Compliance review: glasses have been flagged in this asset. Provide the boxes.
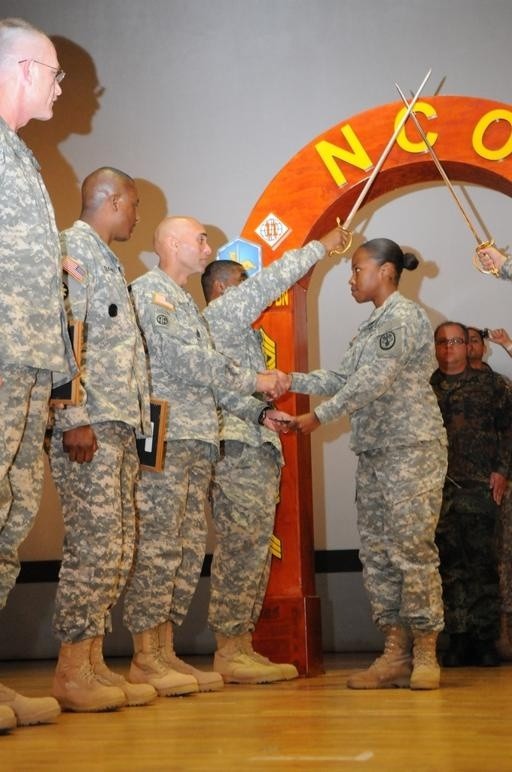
[436,337,466,346]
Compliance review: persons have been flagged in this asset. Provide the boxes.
[203,228,357,685]
[263,238,448,689]
[432,321,512,667]
[53,166,156,713]
[468,327,512,659]
[0,15,60,732]
[477,247,512,286]
[130,215,288,695]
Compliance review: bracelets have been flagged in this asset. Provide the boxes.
[259,409,266,424]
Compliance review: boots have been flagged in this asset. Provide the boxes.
[127,623,199,697]
[0,684,61,732]
[212,631,282,686]
[47,640,127,712]
[407,626,442,692]
[345,622,413,690]
[89,636,158,707]
[239,629,300,681]
[154,619,225,692]
